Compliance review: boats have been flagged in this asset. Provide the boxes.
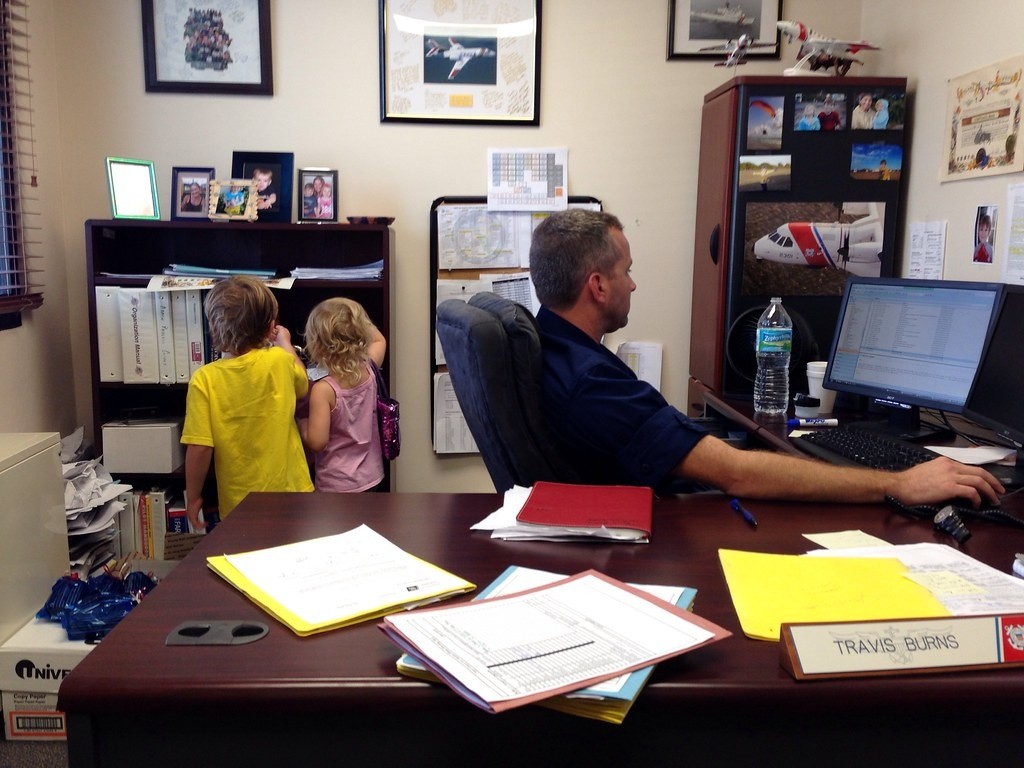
[690,0,755,25]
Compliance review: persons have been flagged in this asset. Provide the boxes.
[852,93,889,129]
[973,215,992,263]
[296,296,386,493]
[250,166,276,209]
[179,275,315,533]
[878,159,890,180]
[303,174,333,219]
[798,104,820,130]
[181,181,206,212]
[530,209,1005,503]
[818,99,841,130]
[223,185,244,214]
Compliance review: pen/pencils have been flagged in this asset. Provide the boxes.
[731,499,758,527]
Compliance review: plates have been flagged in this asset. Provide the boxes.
[346,215,396,227]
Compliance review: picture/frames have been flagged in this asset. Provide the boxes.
[666,0,782,60]
[140,1,274,94]
[105,150,338,223]
[380,0,543,125]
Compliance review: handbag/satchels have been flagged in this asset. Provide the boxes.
[368,361,400,460]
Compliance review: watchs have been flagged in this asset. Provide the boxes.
[934,505,973,545]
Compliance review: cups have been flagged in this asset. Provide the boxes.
[806,360,837,414]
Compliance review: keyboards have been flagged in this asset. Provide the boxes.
[789,424,937,471]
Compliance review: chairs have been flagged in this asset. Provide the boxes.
[437,293,549,492]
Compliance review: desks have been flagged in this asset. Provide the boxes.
[1,433,72,646]
[59,388,1024,768]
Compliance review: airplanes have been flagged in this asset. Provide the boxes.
[698,34,774,68]
[426,37,495,80]
[775,21,883,75]
[751,200,884,278]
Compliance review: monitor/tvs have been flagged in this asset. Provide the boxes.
[822,275,1024,489]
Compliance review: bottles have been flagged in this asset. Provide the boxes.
[754,297,793,415]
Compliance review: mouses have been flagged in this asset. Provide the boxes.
[936,487,1001,511]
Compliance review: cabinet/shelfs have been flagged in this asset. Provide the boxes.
[85,218,390,506]
[687,76,910,419]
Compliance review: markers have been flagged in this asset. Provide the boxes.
[787,418,838,427]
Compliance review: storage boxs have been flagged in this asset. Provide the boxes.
[0,617,101,741]
[102,421,185,475]
